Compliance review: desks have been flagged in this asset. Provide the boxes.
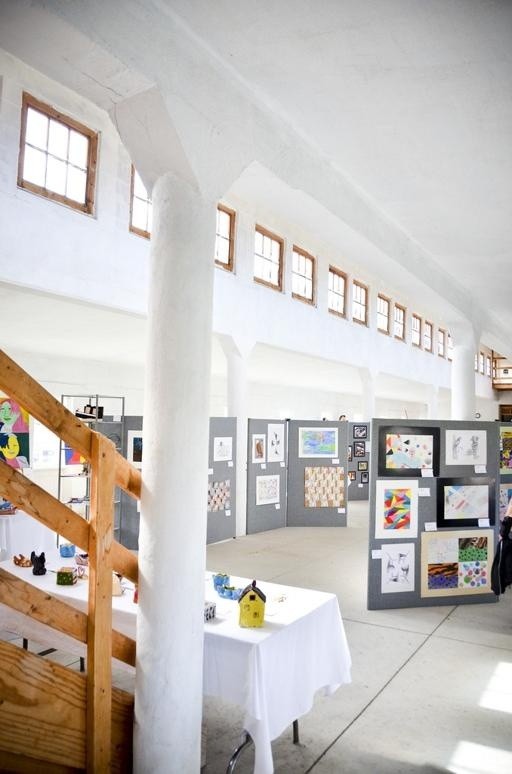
[0,542,355,774]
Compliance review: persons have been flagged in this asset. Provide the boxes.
[1,433,29,468]
[0,396,29,432]
